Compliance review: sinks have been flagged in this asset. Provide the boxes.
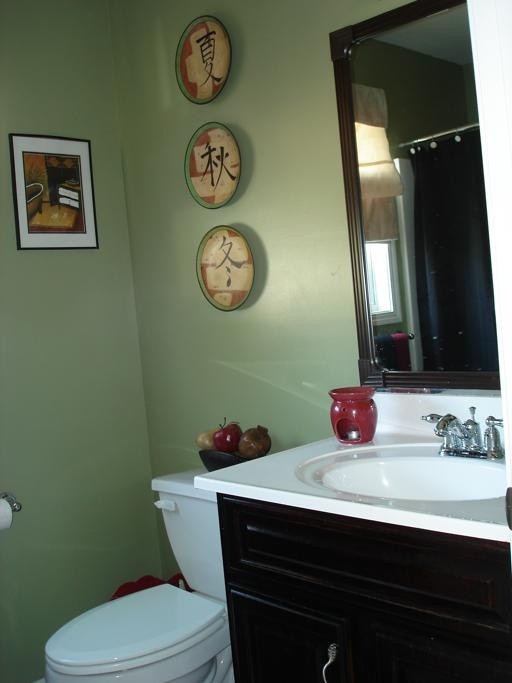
[295,443,510,501]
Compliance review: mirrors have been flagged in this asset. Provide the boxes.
[328,0,499,392]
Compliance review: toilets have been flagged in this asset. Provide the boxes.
[41,465,236,683]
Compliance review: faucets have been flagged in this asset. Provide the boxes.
[433,414,482,451]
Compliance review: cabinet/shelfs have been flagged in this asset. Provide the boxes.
[214,495,511,683]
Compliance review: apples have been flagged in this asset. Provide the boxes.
[213,415,243,453]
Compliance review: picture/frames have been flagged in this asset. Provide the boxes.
[9,132,99,250]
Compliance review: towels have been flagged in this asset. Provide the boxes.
[375,331,410,371]
